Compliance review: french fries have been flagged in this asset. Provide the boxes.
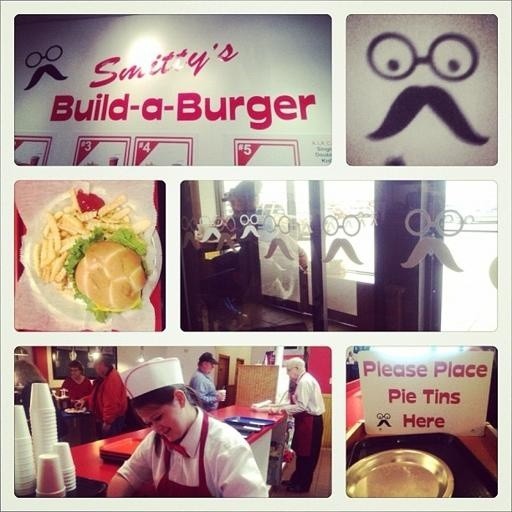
[33,188,131,290]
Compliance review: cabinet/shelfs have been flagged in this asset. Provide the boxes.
[246,411,296,498]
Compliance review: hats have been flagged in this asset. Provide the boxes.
[118,356,184,401]
[198,352,220,364]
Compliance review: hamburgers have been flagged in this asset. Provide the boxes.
[65,226,148,323]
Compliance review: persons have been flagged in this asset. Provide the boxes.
[63,358,93,412]
[77,354,128,437]
[283,220,309,276]
[208,181,259,329]
[189,352,226,410]
[267,356,326,493]
[108,357,269,497]
[15,360,68,439]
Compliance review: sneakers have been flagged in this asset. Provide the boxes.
[280,479,310,493]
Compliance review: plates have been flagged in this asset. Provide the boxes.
[23,187,164,325]
[346,447,456,497]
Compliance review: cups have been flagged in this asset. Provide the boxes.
[217,390,227,402]
[14,382,77,498]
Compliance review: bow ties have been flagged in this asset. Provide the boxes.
[160,436,189,457]
